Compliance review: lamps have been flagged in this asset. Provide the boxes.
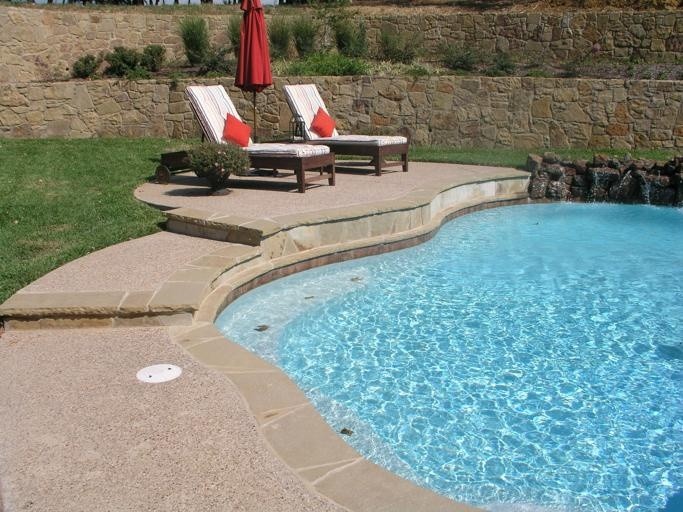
[289,109,306,143]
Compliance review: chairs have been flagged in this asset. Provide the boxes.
[155,85,335,193]
[283,84,408,176]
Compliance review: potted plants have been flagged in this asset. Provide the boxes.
[187,143,252,195]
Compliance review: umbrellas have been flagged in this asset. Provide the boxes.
[235,1,273,143]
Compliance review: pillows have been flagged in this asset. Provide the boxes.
[224,113,253,147]
[312,106,337,138]
